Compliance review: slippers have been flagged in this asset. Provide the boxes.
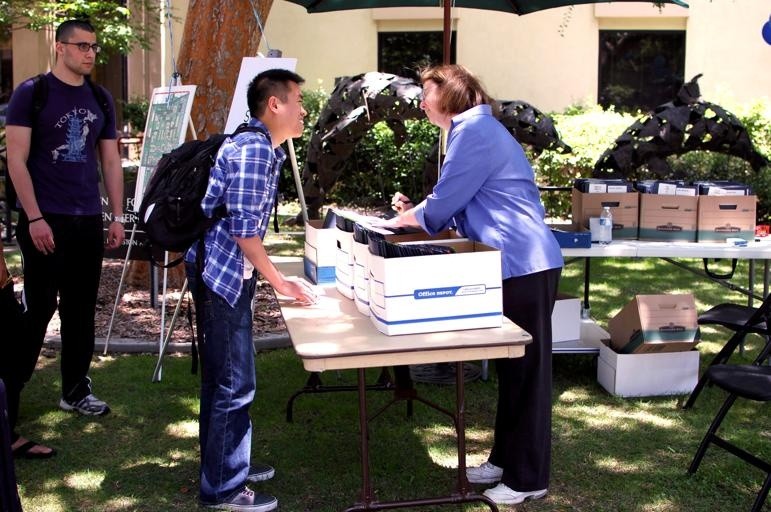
[12,440,58,460]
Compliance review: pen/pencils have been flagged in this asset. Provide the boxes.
[373,200,414,211]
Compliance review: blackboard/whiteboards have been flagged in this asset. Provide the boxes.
[99,168,164,261]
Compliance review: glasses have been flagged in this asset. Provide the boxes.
[60,41,99,54]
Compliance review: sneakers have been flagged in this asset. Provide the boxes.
[482,481,549,506]
[465,461,504,485]
[199,486,280,512]
[57,392,112,417]
[245,462,277,483]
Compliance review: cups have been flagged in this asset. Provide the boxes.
[755,225,770,237]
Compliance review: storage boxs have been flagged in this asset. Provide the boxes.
[352,230,468,315]
[698,196,758,243]
[608,293,702,353]
[573,187,638,240]
[633,190,697,243]
[596,337,700,398]
[333,226,353,300]
[300,211,336,286]
[550,290,583,344]
[367,238,504,337]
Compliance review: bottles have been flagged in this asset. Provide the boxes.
[598,206,612,246]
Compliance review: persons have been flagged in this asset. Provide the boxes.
[0,221,56,459]
[6,21,125,416]
[368,61,566,504]
[186,69,319,512]
[0,377,23,512]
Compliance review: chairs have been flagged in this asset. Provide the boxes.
[681,294,771,409]
[685,337,771,512]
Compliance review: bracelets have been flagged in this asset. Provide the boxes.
[111,216,124,224]
[28,217,44,223]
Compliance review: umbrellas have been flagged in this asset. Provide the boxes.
[285,0,691,178]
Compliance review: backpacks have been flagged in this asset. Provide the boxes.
[5,73,111,213]
[135,123,278,270]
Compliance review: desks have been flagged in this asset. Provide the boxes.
[553,230,771,368]
[269,252,535,512]
